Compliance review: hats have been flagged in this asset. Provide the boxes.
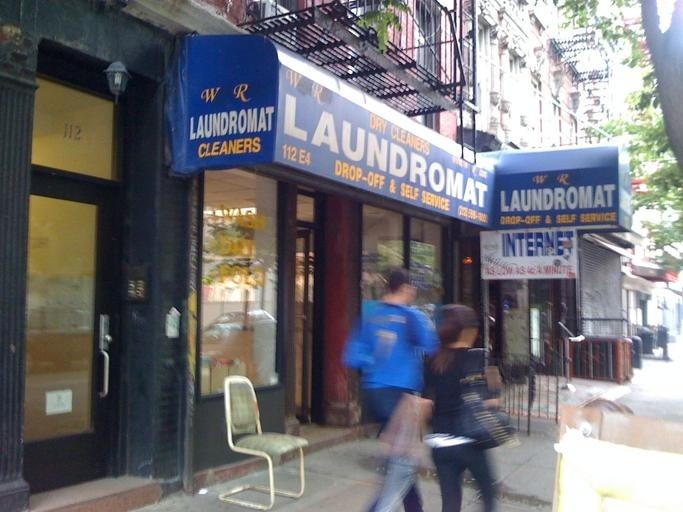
[436,303,479,325]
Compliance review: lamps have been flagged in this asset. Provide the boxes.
[105,60,132,103]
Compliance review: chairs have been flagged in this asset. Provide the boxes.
[219,374,309,510]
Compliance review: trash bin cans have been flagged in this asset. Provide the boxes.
[633,325,669,368]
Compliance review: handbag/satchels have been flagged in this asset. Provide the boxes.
[456,347,515,450]
